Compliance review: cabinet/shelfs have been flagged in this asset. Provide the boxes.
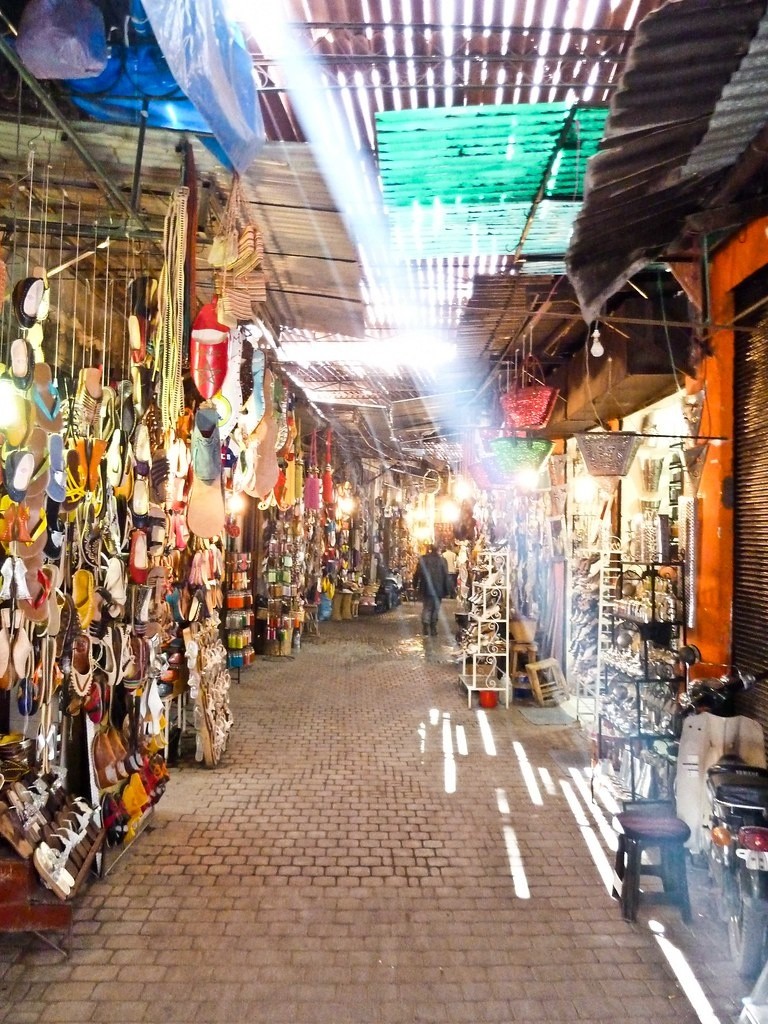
[458,546,511,711]
[590,549,689,812]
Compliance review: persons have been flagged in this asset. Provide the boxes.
[442,545,457,599]
[412,544,449,635]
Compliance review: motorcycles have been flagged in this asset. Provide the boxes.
[381,564,406,611]
[656,645,768,981]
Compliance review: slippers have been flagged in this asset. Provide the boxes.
[1,278,235,903]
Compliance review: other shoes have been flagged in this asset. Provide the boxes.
[458,564,504,654]
[431,622,438,635]
[422,623,429,635]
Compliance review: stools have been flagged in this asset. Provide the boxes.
[525,657,571,708]
[612,811,694,926]
[510,642,541,697]
